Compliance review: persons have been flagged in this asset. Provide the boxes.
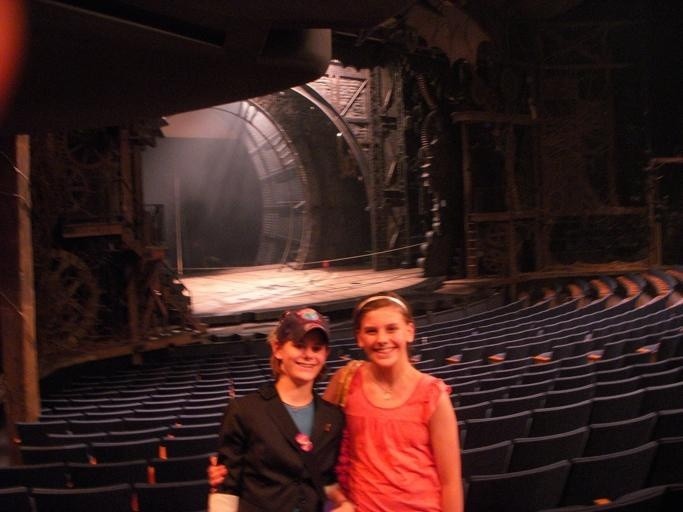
[206,305,357,511]
[205,288,465,512]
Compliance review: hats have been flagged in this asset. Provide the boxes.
[276,308,331,346]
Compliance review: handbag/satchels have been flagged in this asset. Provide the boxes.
[326,359,367,511]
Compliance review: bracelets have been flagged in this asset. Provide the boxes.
[338,499,351,505]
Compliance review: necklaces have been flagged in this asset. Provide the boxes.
[371,370,410,399]
